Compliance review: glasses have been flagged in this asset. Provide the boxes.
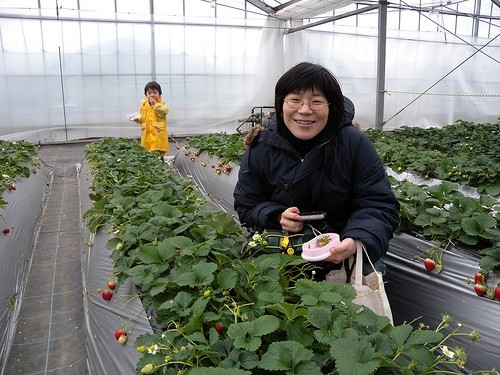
[281,98,334,111]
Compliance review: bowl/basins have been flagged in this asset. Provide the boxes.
[127,112,142,123]
[301,233,342,261]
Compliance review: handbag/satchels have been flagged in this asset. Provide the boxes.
[326,238,394,330]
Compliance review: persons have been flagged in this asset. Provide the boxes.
[233,61,399,309]
[129,80,170,165]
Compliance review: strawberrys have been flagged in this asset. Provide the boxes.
[474,271,500,301]
[136,345,147,353]
[108,280,116,290]
[184,144,231,174]
[140,364,154,375]
[10,185,16,191]
[423,257,435,271]
[396,137,475,191]
[118,335,126,344]
[102,289,112,300]
[86,200,125,252]
[215,322,223,332]
[115,330,125,341]
[3,228,10,234]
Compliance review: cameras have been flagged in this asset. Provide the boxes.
[291,211,327,222]
